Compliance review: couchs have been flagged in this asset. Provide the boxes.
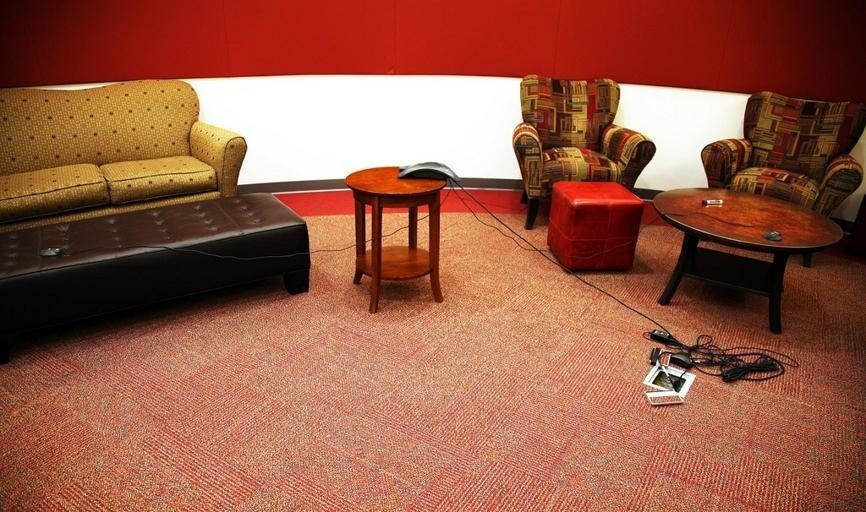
[701,89,866,269]
[512,74,656,230]
[1,78,248,234]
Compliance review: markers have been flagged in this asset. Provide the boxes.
[703,200,723,205]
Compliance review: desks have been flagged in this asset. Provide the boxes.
[345,165,448,314]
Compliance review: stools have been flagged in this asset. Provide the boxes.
[548,181,645,271]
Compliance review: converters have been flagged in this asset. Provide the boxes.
[651,329,672,343]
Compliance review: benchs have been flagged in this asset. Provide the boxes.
[1,192,311,368]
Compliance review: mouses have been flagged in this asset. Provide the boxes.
[670,352,693,369]
